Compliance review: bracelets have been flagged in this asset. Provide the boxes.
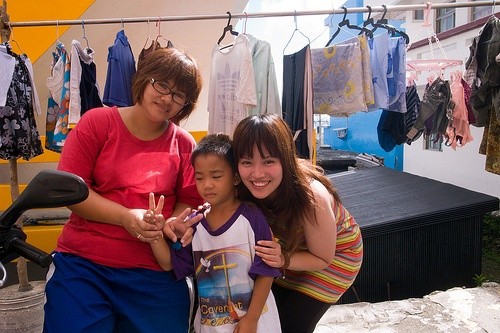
[281,249,290,272]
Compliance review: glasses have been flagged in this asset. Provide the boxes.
[150,78,189,106]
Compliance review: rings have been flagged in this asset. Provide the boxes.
[137,234,141,239]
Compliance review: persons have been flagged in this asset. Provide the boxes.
[232,112,364,332]
[142,132,283,333]
[40,47,203,333]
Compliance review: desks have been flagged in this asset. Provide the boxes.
[325,167,500,303]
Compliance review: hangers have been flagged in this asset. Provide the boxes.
[53,17,89,52]
[283,4,409,55]
[217,12,248,50]
[120,16,169,48]
[7,22,23,53]
[478,0,496,44]
